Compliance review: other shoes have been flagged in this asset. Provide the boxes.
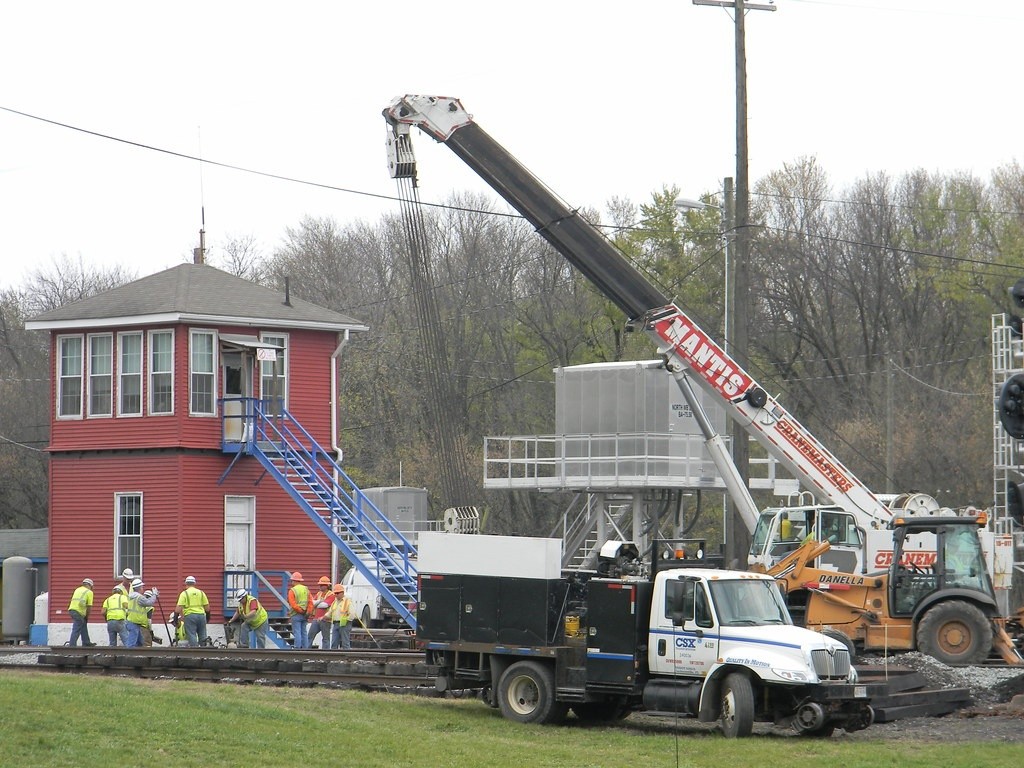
[86,643,95,647]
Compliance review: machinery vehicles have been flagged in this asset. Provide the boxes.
[383,95,1023,668]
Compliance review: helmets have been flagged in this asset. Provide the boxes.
[186,576,195,582]
[334,584,344,592]
[317,576,332,585]
[132,579,145,588]
[235,589,248,602]
[122,568,134,579]
[144,590,152,597]
[113,585,123,595]
[289,572,304,581]
[82,579,93,587]
[168,612,181,623]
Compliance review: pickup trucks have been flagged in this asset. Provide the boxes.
[340,561,410,631]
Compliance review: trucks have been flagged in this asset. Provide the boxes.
[417,531,875,738]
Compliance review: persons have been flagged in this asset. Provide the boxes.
[227,589,268,649]
[127,579,159,646]
[102,586,128,646]
[168,612,186,642]
[69,579,97,646]
[143,590,154,633]
[775,513,791,539]
[113,567,134,596]
[288,572,312,647]
[316,584,355,648]
[308,576,335,649]
[175,576,210,643]
[781,517,836,561]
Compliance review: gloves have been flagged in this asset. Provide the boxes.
[152,586,160,596]
[206,611,211,624]
[301,611,307,619]
[173,613,179,622]
[223,621,231,626]
